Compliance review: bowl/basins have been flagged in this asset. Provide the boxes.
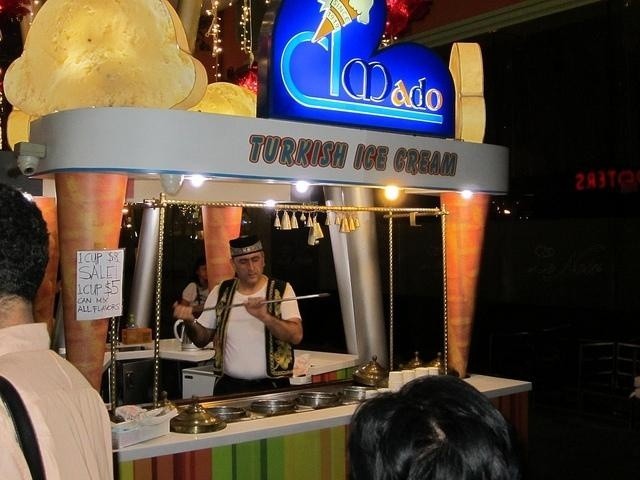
[252,398,297,416]
[297,392,338,408]
[343,386,378,400]
[205,405,246,423]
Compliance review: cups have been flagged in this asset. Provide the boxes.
[174,318,203,351]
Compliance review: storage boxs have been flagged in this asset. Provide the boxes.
[122,328,152,345]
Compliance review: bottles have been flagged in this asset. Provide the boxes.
[126,313,138,328]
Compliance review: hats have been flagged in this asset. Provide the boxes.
[229,237,263,257]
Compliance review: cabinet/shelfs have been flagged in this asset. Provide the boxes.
[159,356,192,399]
[116,357,155,400]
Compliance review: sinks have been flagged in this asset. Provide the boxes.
[115,345,154,352]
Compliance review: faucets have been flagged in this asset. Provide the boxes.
[115,336,121,346]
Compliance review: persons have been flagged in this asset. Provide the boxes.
[346,374,522,480]
[180,256,210,317]
[173,235,304,396]
[0,183,115,480]
